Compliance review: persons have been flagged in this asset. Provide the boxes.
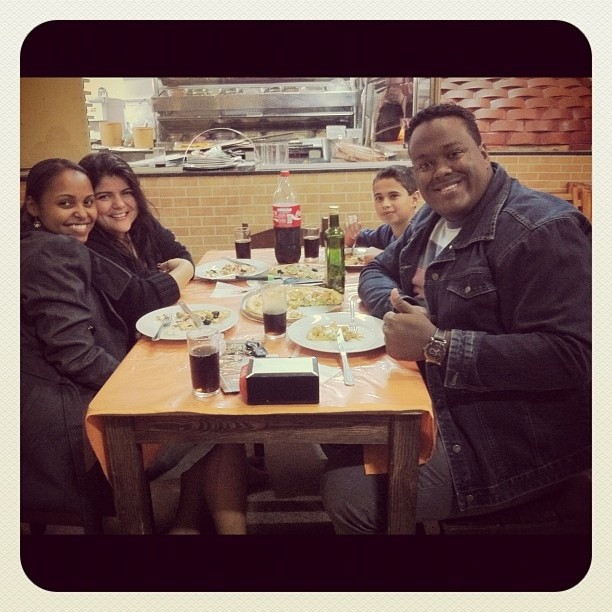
[345,165,421,266]
[320,102,592,535]
[78,153,195,342]
[20,157,248,536]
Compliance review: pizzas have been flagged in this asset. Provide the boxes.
[245,284,343,320]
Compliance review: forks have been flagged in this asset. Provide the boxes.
[151,314,171,340]
[348,302,358,335]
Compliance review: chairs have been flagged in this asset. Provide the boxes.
[241,219,327,250]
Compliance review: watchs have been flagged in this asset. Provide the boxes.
[422,328,449,367]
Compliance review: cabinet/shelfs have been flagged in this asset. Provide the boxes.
[152,77,356,149]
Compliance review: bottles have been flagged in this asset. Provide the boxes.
[324,215,344,294]
[273,171,301,264]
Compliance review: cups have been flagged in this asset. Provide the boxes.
[187,328,221,398]
[303,226,319,262]
[262,282,286,339]
[234,227,251,259]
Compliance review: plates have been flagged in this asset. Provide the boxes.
[343,247,386,268]
[240,284,341,323]
[287,312,389,354]
[195,259,272,281]
[270,263,326,284]
[136,303,239,341]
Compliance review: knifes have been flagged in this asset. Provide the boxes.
[336,328,353,386]
[179,301,203,329]
